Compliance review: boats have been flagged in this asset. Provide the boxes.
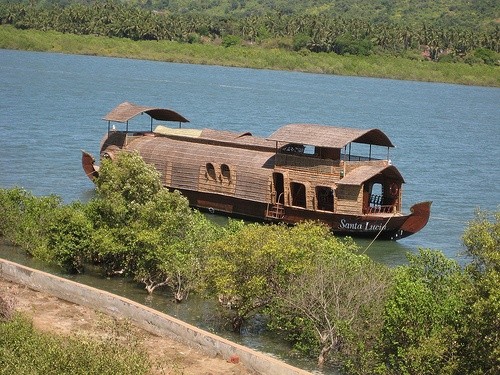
[82,102,432,246]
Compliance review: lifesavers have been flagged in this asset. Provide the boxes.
[388,183,398,196]
